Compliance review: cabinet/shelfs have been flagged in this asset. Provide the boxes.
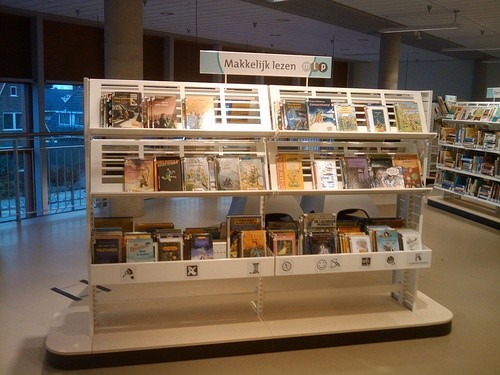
[83,77,438,338]
[427,102,500,231]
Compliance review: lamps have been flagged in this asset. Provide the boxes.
[441,38,500,52]
[377,10,460,33]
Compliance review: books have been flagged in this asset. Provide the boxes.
[91,213,422,265]
[427,87,500,202]
[122,152,423,193]
[96,93,252,130]
[276,97,424,132]
